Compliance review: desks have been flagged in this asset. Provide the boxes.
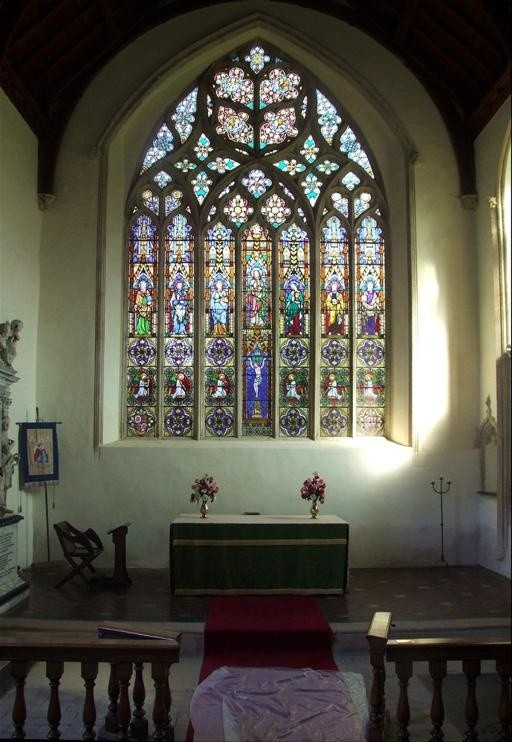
[170,514,349,601]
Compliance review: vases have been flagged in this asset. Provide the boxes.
[200,499,209,519]
[311,502,319,519]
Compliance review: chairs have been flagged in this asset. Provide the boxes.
[53,520,104,592]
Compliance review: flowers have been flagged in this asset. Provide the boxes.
[190,472,219,503]
[300,471,327,503]
